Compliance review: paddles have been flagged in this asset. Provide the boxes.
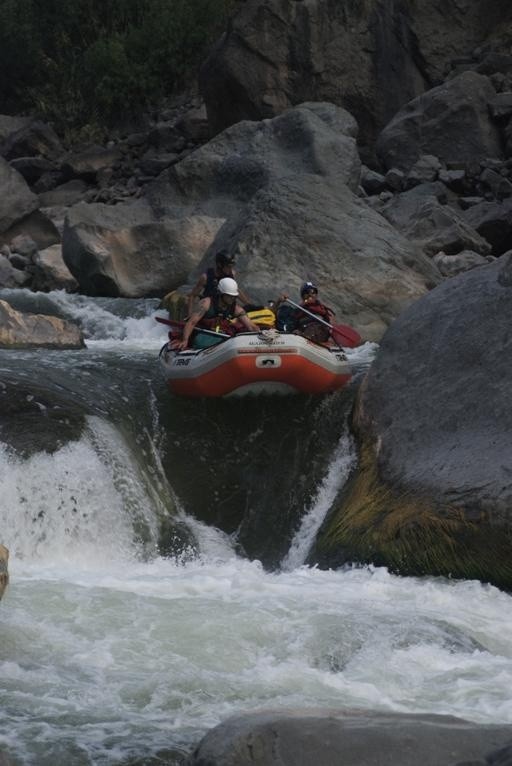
[155,317,230,339]
[285,298,361,348]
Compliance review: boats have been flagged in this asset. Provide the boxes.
[158,333,363,404]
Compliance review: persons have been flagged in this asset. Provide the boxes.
[185,250,255,321]
[166,276,263,350]
[270,281,337,346]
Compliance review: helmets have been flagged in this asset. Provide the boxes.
[215,248,237,267]
[300,281,318,297]
[217,277,240,297]
